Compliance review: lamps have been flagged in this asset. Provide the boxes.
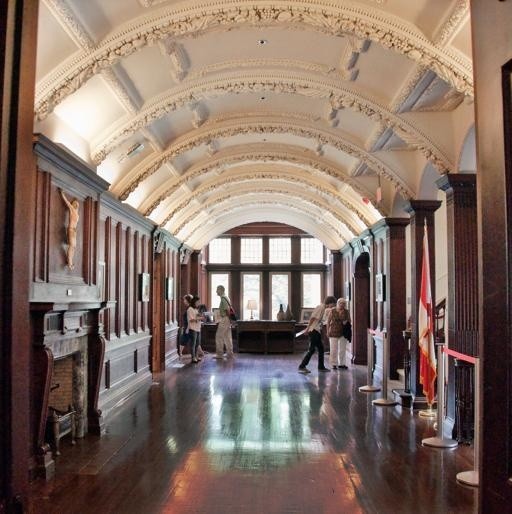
[246,299,259,319]
[376,175,382,202]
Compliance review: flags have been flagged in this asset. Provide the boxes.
[415,225,437,406]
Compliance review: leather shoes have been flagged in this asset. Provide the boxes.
[192,356,201,364]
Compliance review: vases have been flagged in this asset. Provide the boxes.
[286,303,294,321]
[277,304,286,320]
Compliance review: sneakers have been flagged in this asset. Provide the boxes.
[213,354,231,359]
[318,366,348,372]
[298,367,311,373]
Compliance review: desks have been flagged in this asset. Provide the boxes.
[200,319,329,354]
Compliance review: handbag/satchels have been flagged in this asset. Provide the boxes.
[226,306,238,321]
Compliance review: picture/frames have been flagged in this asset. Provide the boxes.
[165,277,176,300]
[212,307,221,322]
[140,272,151,303]
[345,281,350,301]
[300,308,316,324]
[375,273,384,302]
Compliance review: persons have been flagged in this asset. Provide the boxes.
[298,297,337,373]
[58,188,80,270]
[179,293,204,356]
[212,285,236,359]
[187,296,205,363]
[326,298,351,369]
